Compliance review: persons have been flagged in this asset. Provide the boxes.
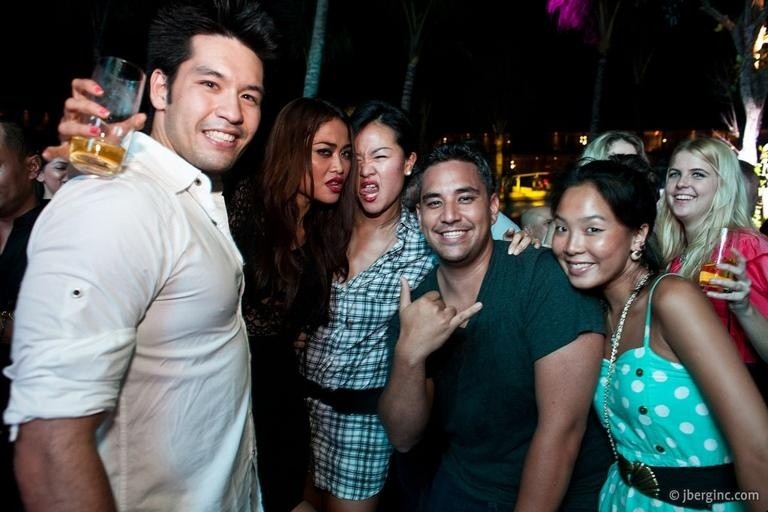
[2,2,768,512]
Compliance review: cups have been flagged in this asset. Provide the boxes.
[67,52,147,181]
[698,225,739,295]
